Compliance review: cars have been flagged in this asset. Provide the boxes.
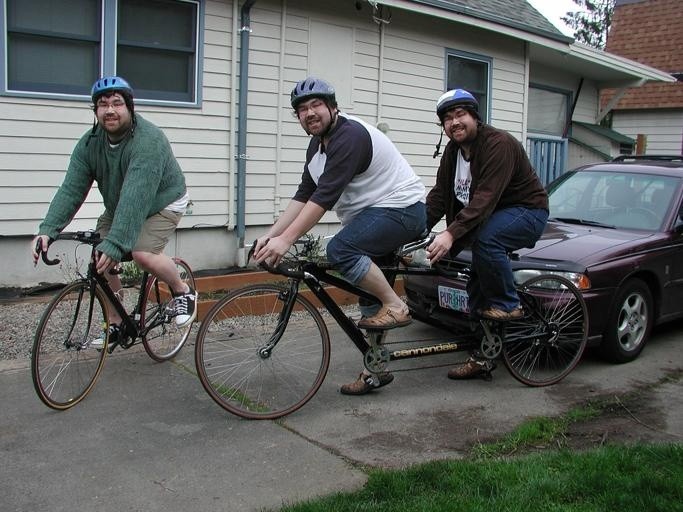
[402,153,683,364]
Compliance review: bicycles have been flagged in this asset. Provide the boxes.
[193,238,589,421]
[29,228,195,412]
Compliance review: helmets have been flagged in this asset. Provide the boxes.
[91,77,134,111]
[291,77,335,108]
[435,89,479,119]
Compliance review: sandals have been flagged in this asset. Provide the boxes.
[341,372,394,395]
[357,307,412,329]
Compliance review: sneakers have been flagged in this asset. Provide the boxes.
[172,283,199,329]
[448,357,497,379]
[90,323,122,350]
[481,306,525,321]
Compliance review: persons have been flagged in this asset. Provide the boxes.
[428,88,550,378]
[33,76,199,350]
[251,77,427,398]
